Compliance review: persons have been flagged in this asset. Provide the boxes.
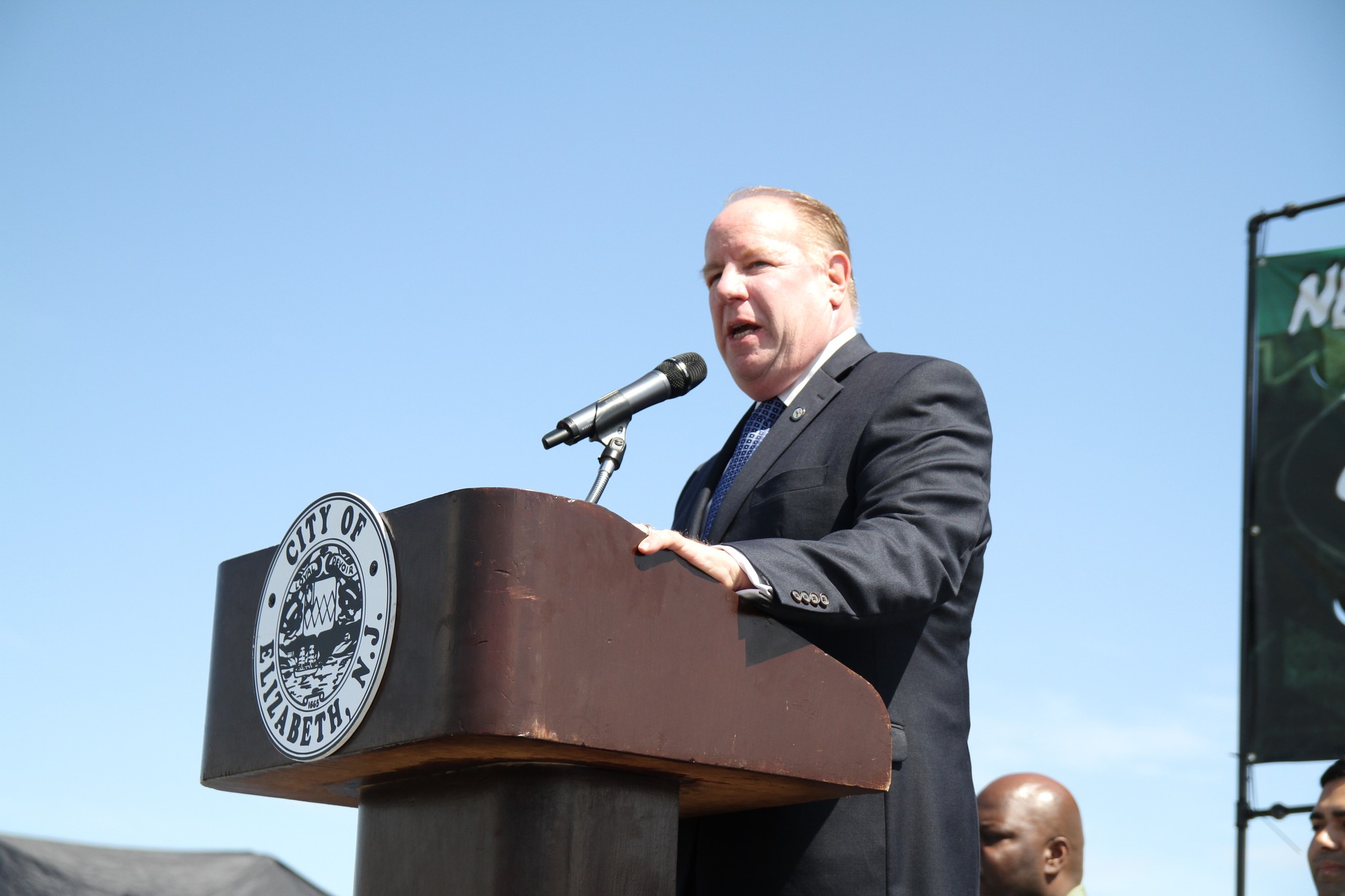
[977,772,1091,896]
[1306,758,1345,896]
[638,185,992,896]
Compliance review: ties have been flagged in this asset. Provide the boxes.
[702,395,787,542]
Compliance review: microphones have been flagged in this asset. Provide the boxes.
[541,352,708,450]
